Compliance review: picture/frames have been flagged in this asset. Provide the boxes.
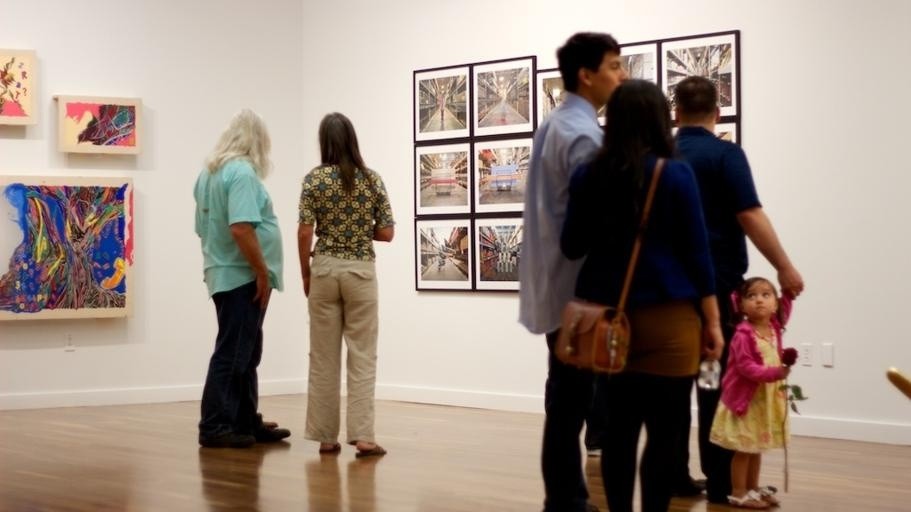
[0,49,143,321]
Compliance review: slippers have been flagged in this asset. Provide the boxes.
[355,444,386,458]
[318,442,340,453]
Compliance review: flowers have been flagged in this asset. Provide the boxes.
[779,347,808,491]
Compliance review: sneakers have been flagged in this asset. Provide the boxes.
[729,486,780,508]
[675,478,707,495]
[586,449,601,456]
[198,417,292,448]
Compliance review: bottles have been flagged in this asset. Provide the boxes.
[696,354,722,390]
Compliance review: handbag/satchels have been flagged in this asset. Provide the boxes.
[555,300,630,373]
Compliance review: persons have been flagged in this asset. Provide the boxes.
[306,459,379,512]
[297,111,396,457]
[668,75,804,504]
[559,78,725,512]
[197,448,289,512]
[708,276,796,509]
[518,32,631,512]
[192,108,291,445]
